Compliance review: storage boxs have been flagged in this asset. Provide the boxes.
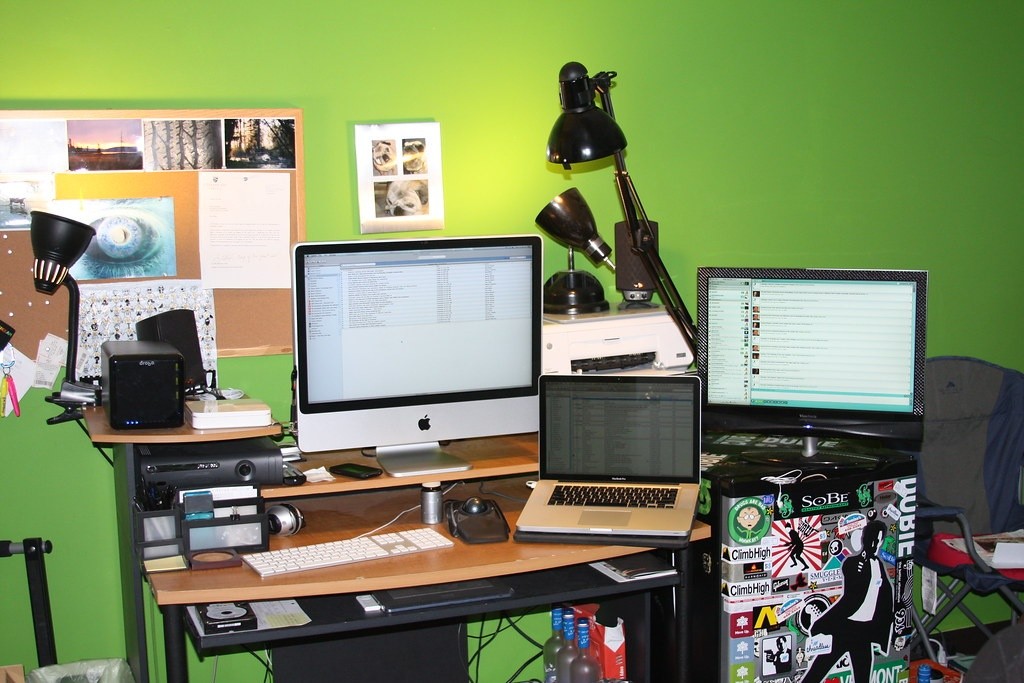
[563,601,626,683]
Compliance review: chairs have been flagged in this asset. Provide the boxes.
[908,355,1024,662]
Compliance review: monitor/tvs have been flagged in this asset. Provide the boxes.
[693,267,928,468]
[289,232,543,477]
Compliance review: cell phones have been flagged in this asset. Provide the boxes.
[329,464,383,479]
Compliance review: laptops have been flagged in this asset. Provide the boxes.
[515,374,702,537]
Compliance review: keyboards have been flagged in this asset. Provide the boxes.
[242,527,455,577]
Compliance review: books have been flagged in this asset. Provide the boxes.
[184,398,272,429]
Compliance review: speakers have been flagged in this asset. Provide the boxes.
[101,341,191,430]
[136,309,207,391]
[614,220,661,309]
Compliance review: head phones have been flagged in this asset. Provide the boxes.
[265,503,310,535]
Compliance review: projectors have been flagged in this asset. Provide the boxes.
[133,434,283,491]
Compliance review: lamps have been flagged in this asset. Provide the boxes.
[29,211,102,426]
[536,62,697,360]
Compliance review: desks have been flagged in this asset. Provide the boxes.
[689,433,917,683]
[82,398,712,683]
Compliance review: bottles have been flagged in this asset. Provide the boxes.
[918,664,931,683]
[570,618,600,683]
[421,481,443,525]
[543,608,565,683]
[557,608,578,683]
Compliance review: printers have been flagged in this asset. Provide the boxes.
[542,311,696,375]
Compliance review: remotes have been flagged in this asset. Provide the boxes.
[281,461,307,486]
